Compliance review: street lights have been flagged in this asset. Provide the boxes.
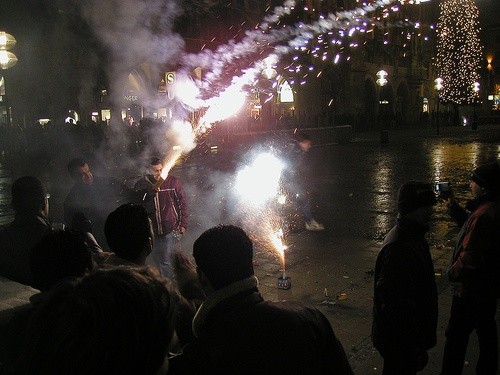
[0,30,17,160]
[434,77,445,137]
[472,81,480,129]
[375,69,388,143]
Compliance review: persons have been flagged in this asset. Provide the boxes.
[370,180,439,375]
[167,224,354,375]
[440,164,500,375]
[0,116,138,128]
[0,158,207,375]
[279,134,324,231]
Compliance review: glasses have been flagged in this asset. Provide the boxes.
[44,192,51,200]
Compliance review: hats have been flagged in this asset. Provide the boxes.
[397,180,438,206]
[470,159,500,195]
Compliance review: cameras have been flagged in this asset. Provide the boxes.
[435,182,450,192]
